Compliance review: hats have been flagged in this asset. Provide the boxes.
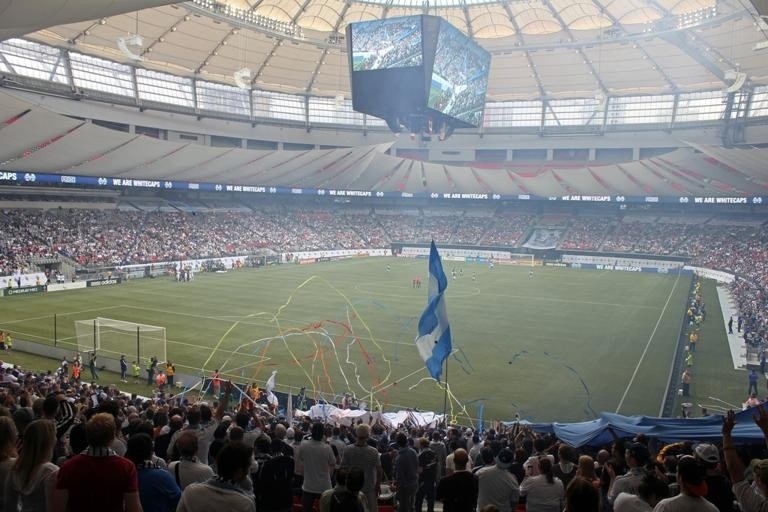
[676,454,709,497]
[624,438,651,465]
[691,440,720,462]
[750,457,767,484]
[495,447,515,469]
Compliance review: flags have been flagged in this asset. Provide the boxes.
[412,240,454,383]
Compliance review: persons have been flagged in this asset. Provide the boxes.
[688,289,768,328]
[1,179,768,292]
[1,328,768,512]
[427,19,489,128]
[348,16,422,73]
